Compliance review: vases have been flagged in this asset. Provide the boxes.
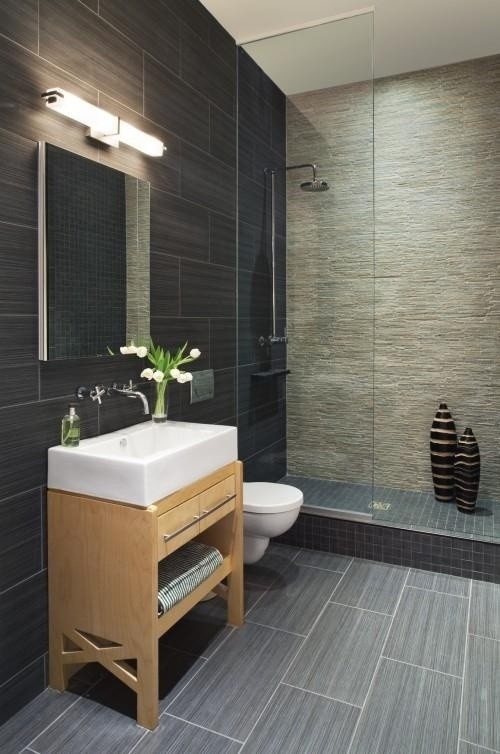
[151,380,171,422]
[430,401,480,515]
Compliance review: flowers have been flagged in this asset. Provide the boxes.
[130,339,203,416]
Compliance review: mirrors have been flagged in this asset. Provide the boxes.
[36,138,153,361]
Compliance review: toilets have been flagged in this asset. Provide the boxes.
[242,480,303,564]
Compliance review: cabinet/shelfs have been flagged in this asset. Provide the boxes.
[48,461,246,731]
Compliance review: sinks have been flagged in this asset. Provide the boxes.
[48,417,238,506]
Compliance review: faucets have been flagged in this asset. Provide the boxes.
[110,387,151,416]
